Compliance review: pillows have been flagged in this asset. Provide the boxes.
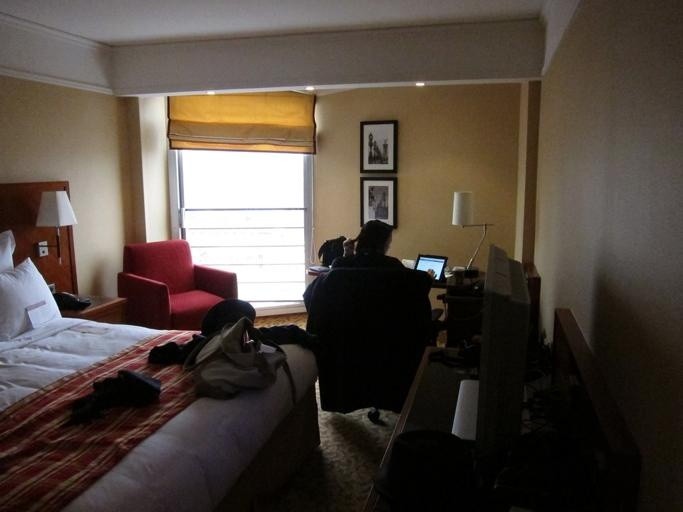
[0,256,62,342]
[0,229,16,273]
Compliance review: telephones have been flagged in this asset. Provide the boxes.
[55,291,91,309]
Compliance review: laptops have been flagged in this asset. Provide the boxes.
[412,254,448,281]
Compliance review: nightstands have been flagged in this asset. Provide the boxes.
[62,294,128,324]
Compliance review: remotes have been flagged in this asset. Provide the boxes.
[474,278,483,291]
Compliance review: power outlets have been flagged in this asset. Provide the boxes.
[38,240,49,257]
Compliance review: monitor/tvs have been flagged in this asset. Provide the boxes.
[450,243,532,450]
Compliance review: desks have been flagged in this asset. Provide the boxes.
[307,261,542,346]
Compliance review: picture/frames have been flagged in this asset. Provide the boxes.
[360,120,399,174]
[360,176,398,230]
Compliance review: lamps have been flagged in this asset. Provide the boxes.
[451,191,496,271]
[36,190,79,265]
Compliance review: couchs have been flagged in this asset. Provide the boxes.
[118,239,238,331]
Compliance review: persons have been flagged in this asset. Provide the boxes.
[343,219,436,292]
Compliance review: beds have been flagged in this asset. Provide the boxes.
[0,318,321,512]
